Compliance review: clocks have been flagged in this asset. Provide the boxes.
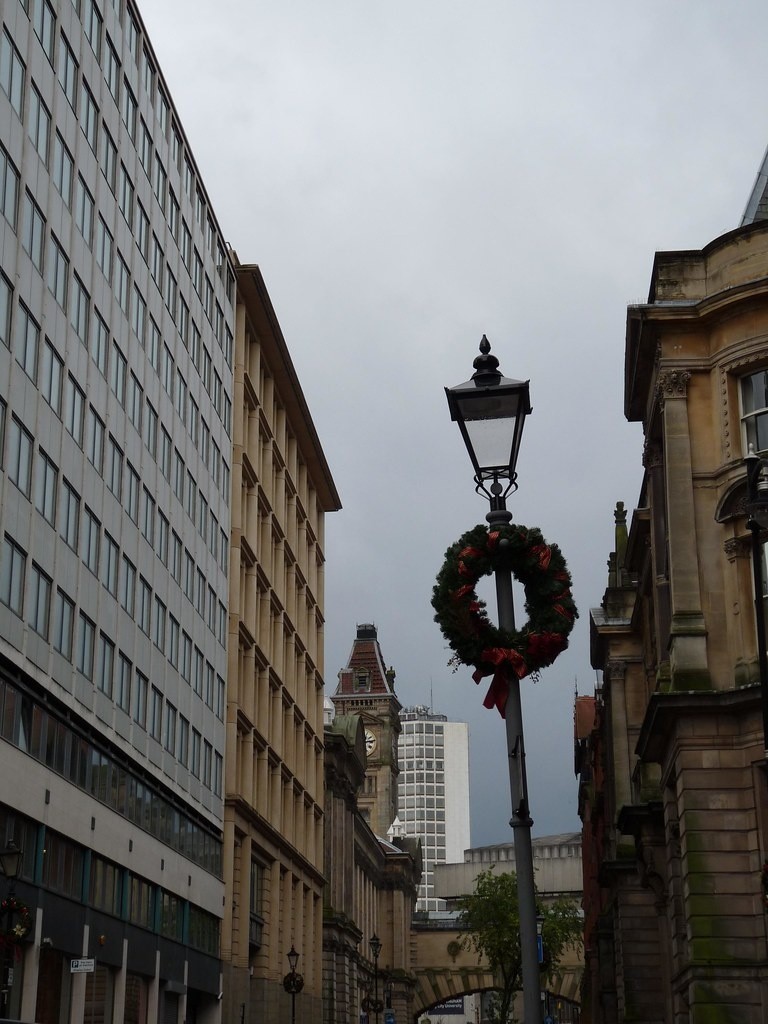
[364,728,377,757]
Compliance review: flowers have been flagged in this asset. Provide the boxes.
[430,523,581,684]
[0,897,32,944]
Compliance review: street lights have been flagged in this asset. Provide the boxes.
[286,943,301,1024]
[370,931,384,1024]
[440,335,548,1024]
